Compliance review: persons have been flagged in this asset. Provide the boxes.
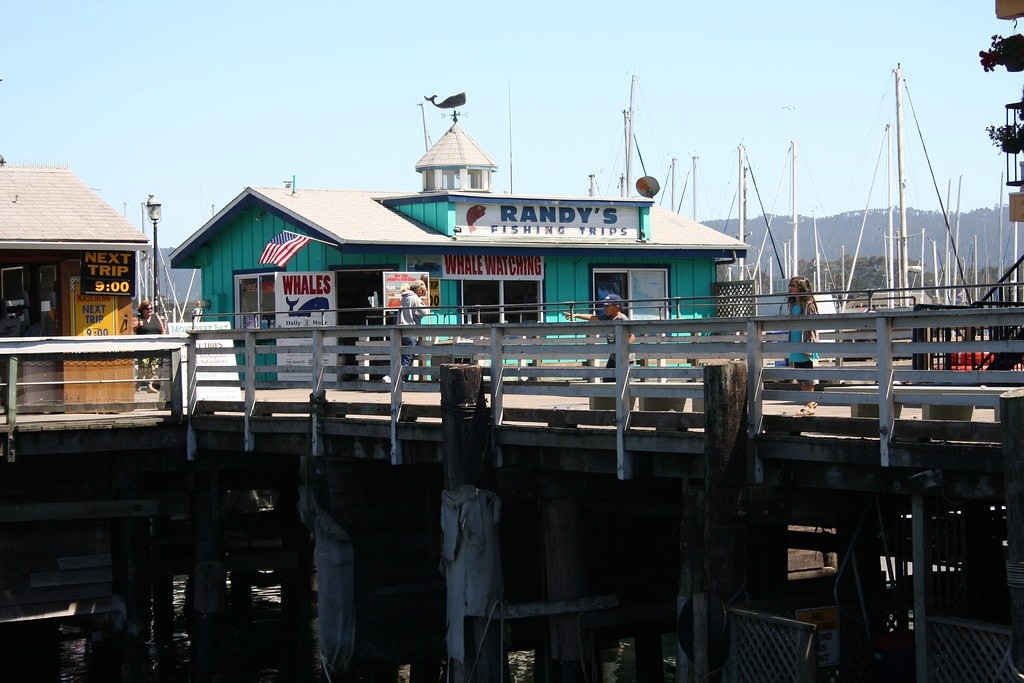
[787,276,819,415]
[399,280,431,383]
[564,294,636,382]
[132,299,167,393]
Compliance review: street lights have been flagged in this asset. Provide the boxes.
[144,191,165,318]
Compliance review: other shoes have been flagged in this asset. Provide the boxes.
[382,375,392,384]
[135,384,141,392]
[147,389,157,393]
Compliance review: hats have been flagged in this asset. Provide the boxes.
[410,284,422,290]
[604,294,623,306]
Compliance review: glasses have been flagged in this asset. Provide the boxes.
[144,307,152,310]
[788,284,798,290]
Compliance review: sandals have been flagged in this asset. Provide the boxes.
[796,403,818,415]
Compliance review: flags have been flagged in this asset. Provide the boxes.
[257,232,311,269]
